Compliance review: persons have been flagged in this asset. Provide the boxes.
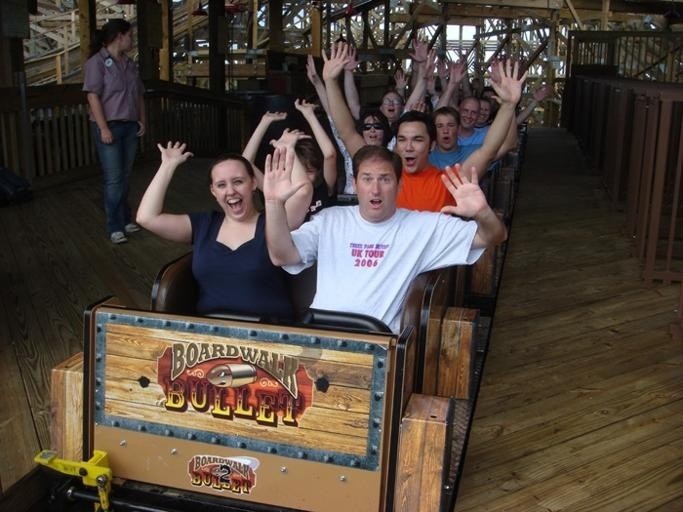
[79,18,145,244]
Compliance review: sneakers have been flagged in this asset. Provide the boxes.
[111,222,143,244]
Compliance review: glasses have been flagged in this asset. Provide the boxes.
[362,123,384,130]
[382,98,402,105]
